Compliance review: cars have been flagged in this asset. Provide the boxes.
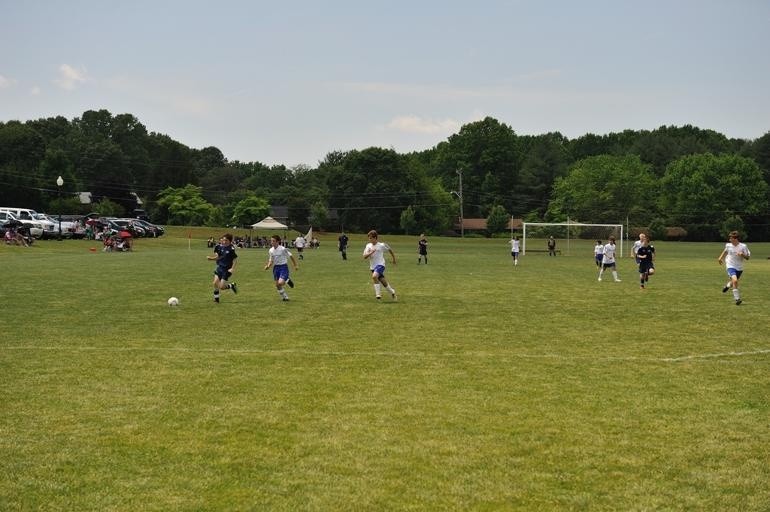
[37,213,164,241]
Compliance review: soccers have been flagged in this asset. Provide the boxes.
[169,297,181,306]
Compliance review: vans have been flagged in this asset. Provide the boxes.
[0,207,55,240]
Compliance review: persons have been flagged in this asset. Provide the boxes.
[594,233,656,290]
[74,215,133,253]
[265,235,298,301]
[416,234,428,265]
[548,236,556,256]
[338,234,349,260]
[207,233,320,261]
[509,236,520,266]
[718,231,751,305]
[363,230,397,302]
[4,218,35,248]
[207,233,238,304]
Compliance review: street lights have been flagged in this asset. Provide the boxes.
[56,175,64,241]
[450,191,464,237]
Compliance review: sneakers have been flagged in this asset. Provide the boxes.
[231,282,239,294]
[735,299,741,305]
[722,286,729,293]
[391,289,397,301]
[286,277,294,288]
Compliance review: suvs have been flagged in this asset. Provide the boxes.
[0,211,43,239]
